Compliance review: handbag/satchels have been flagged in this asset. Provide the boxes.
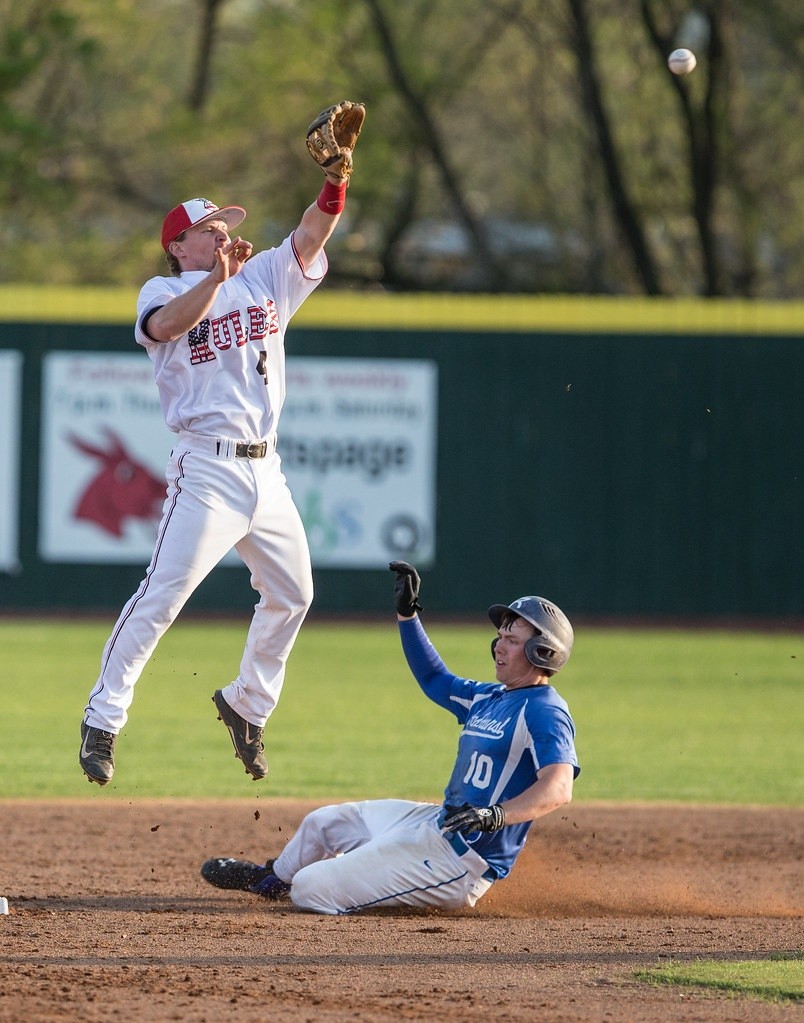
[442,803,506,836]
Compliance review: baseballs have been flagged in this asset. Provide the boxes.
[668,49,697,75]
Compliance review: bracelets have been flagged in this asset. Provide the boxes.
[317,179,348,214]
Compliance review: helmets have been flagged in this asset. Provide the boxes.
[487,595,575,677]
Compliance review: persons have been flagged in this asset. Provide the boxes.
[200,559,578,916]
[79,101,366,786]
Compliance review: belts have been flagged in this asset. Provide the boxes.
[437,811,496,881]
[216,442,267,458]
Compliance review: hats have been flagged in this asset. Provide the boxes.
[160,197,246,251]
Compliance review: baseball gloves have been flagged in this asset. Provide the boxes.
[307,100,365,181]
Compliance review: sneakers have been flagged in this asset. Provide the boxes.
[212,688,270,780]
[201,857,292,901]
[79,717,117,786]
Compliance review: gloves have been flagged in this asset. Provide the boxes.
[388,560,422,617]
